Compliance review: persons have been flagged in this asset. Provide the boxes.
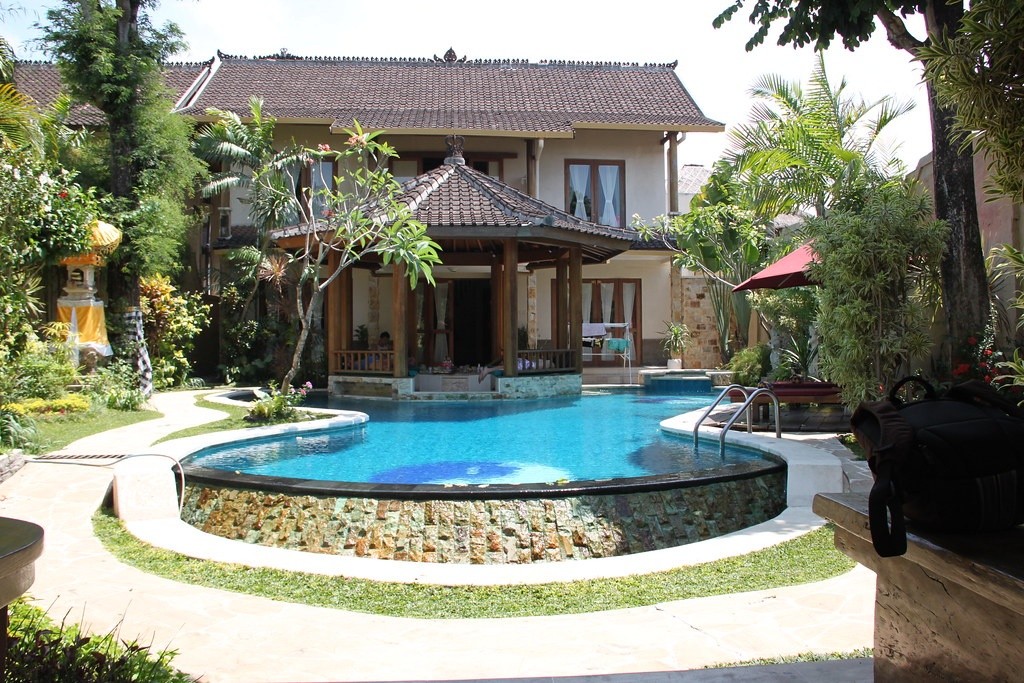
[342,331,415,369]
[478,359,563,382]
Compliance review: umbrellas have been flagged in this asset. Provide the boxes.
[731,238,822,293]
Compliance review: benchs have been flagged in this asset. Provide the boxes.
[728,386,841,421]
[759,381,837,420]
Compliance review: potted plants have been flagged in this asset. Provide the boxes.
[655,320,696,371]
